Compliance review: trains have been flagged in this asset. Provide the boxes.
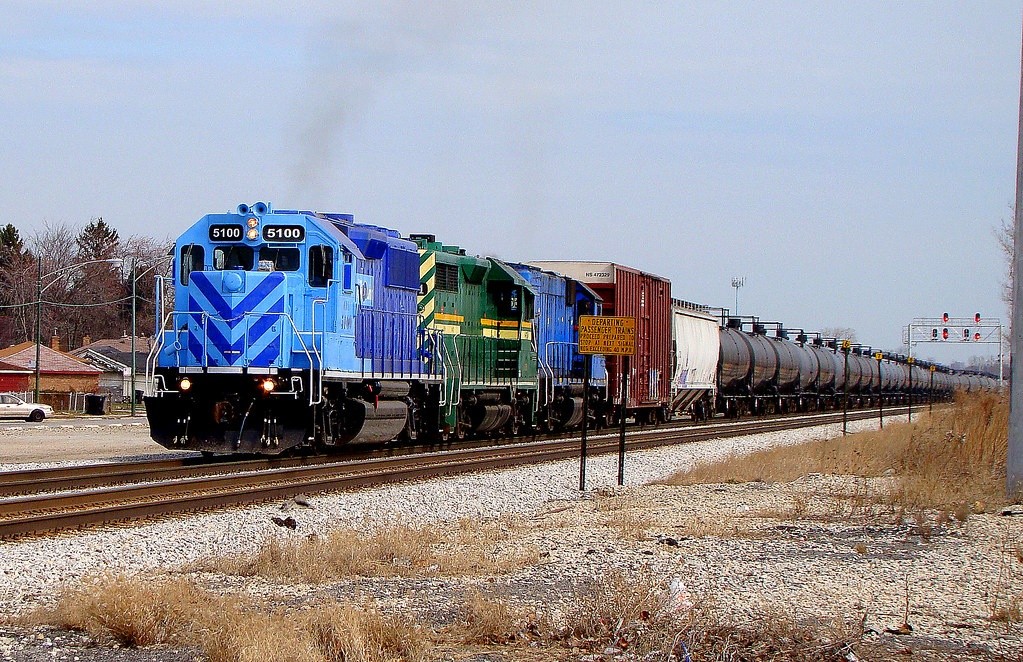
[142,202,1010,461]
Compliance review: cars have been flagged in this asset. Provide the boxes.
[0,393,54,423]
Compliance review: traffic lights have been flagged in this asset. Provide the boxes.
[975,313,980,322]
[944,313,948,322]
[943,329,948,339]
[975,333,980,339]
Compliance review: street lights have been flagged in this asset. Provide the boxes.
[732,277,744,316]
[35,257,123,404]
